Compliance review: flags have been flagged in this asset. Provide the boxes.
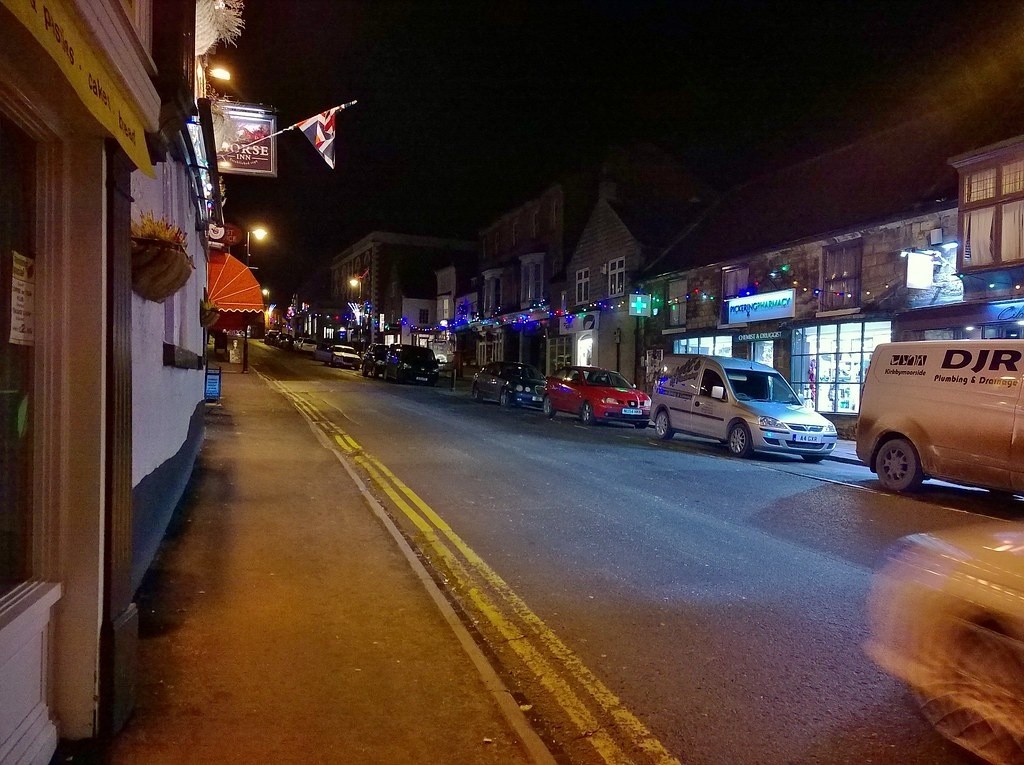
[297,102,344,169]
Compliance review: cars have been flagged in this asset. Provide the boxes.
[541,364,652,429]
[264,330,361,372]
[471,360,549,408]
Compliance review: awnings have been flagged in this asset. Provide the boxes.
[209,250,264,313]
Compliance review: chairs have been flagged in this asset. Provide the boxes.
[595,376,613,385]
[571,373,585,381]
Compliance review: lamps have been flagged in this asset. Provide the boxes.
[916,241,958,272]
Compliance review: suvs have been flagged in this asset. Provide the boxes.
[361,344,391,380]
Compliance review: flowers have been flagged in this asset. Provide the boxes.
[130,210,189,249]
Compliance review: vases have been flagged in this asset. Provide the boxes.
[130,237,194,305]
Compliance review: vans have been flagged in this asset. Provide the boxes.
[649,352,838,462]
[855,339,1024,497]
[383,343,441,385]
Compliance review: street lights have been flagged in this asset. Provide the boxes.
[350,276,364,352]
[241,228,268,374]
